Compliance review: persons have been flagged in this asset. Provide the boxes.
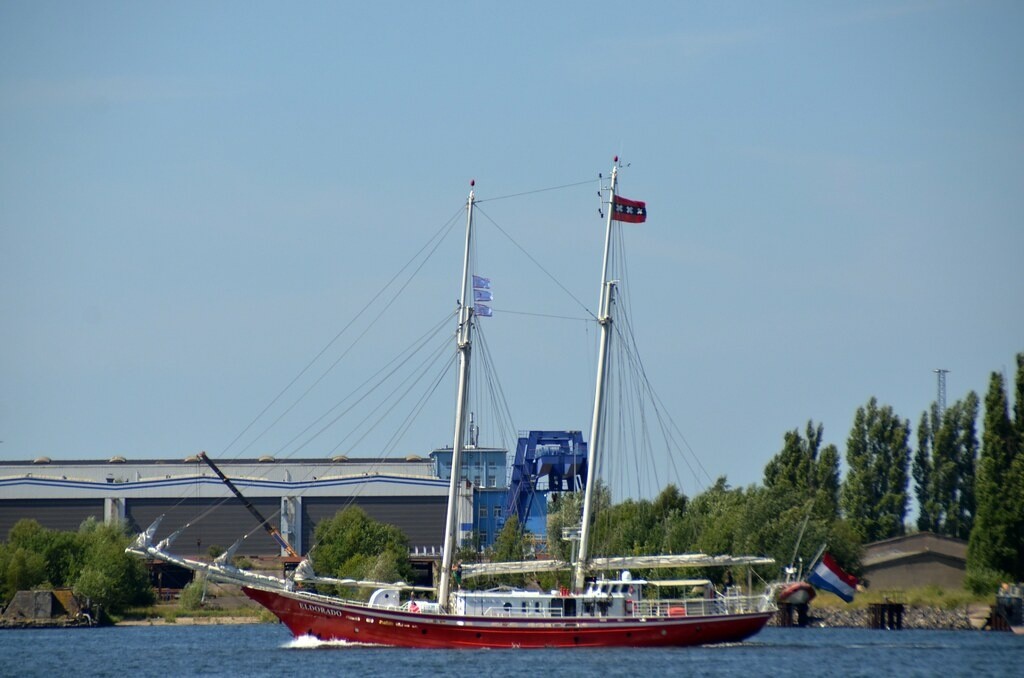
[410,592,419,612]
[451,560,462,586]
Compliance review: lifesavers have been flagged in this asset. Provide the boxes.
[624,599,637,616]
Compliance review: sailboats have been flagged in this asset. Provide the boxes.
[127,154,778,650]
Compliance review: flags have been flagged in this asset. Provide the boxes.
[473,289,492,302]
[612,196,646,224]
[472,276,491,288]
[806,552,857,602]
[474,304,493,316]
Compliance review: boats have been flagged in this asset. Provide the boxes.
[767,580,815,605]
[997,583,1024,626]
